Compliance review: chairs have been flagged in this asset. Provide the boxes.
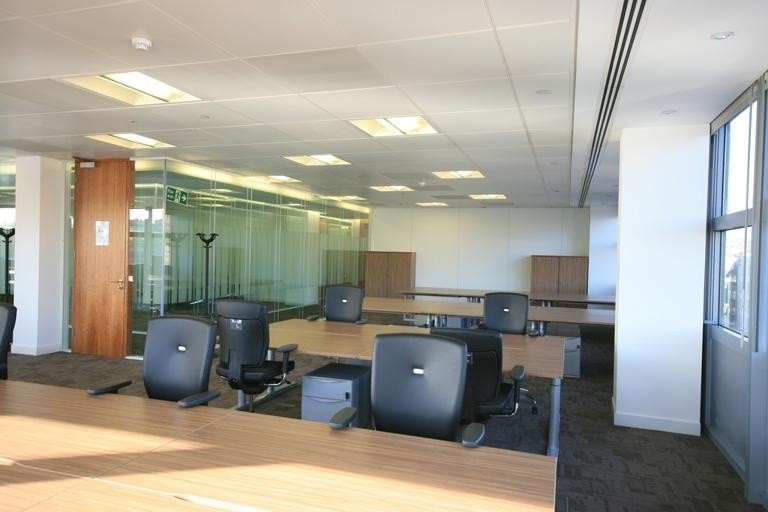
[90,315,218,408]
[212,299,300,412]
[479,291,538,336]
[307,285,362,326]
[429,329,527,446]
[328,333,486,447]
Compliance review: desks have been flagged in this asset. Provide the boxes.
[0,373,558,511]
[361,287,614,333]
[263,317,565,455]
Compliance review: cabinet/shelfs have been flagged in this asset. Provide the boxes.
[530,254,589,309]
[364,251,416,324]
